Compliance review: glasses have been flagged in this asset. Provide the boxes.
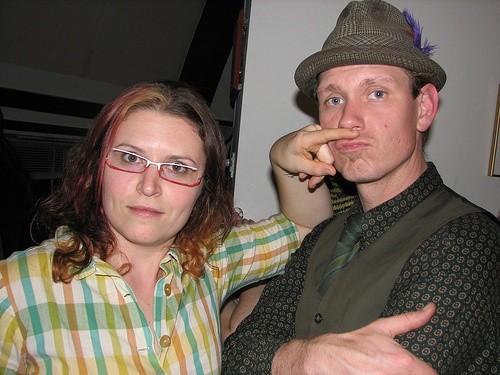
[104,148,204,186]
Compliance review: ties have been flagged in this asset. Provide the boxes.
[320,215,364,295]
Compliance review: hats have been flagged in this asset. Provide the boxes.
[294,0,447,107]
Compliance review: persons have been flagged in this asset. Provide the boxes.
[0,82,360,375]
[219,0,500,375]
